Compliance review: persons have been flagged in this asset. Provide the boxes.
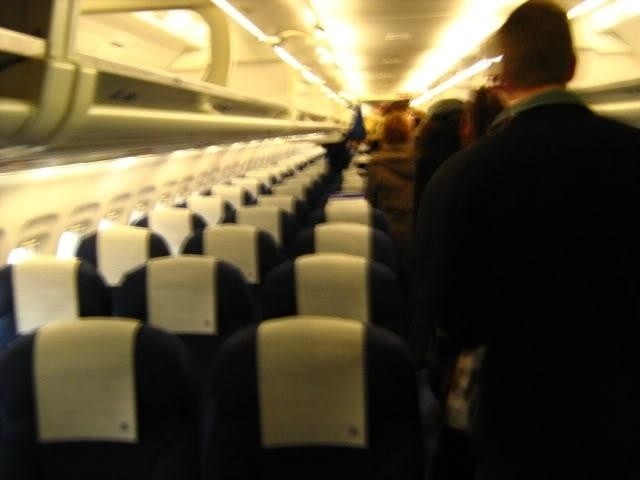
[362,86,511,252]
[420,3,638,479]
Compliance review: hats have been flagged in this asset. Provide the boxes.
[380,99,410,116]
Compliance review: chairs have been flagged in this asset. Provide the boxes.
[0,256,108,350]
[294,221,394,277]
[180,224,278,300]
[223,206,300,259]
[135,205,206,258]
[199,183,257,209]
[175,194,236,232]
[0,316,205,480]
[115,254,261,369]
[248,195,306,225]
[255,253,404,332]
[75,225,171,323]
[205,314,424,479]
[308,197,388,239]
[244,143,372,199]
